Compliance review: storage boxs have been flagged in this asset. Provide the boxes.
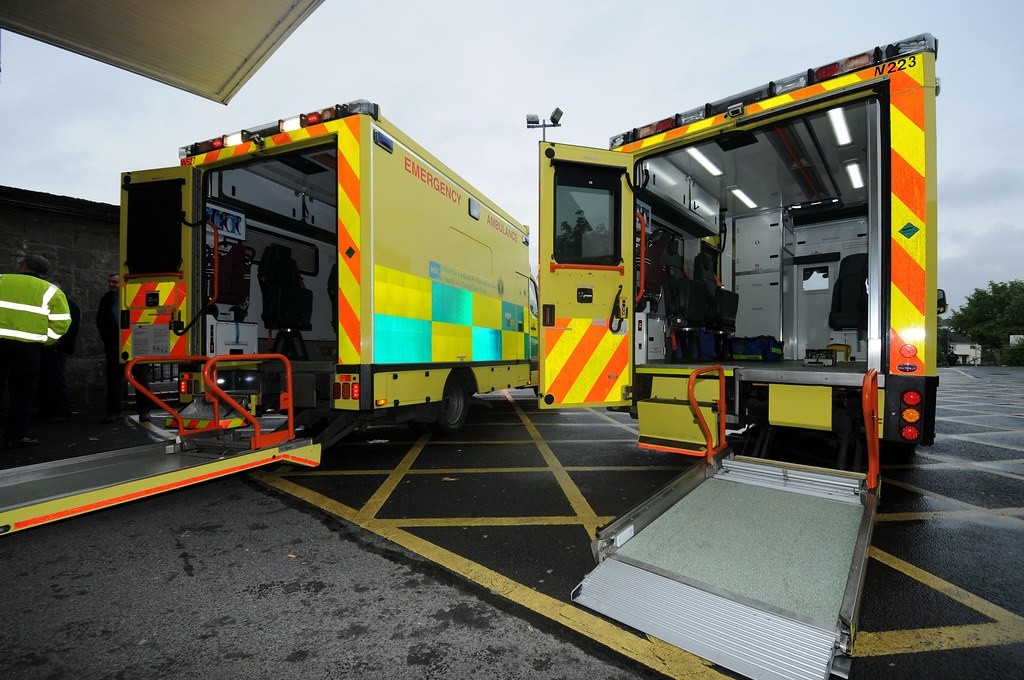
[729,335,781,363]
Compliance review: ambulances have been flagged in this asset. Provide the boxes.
[540,33,947,459]
[117,102,542,435]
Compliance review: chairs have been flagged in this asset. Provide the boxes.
[827,253,869,331]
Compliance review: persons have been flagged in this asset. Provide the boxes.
[94,272,151,423]
[0,253,80,450]
